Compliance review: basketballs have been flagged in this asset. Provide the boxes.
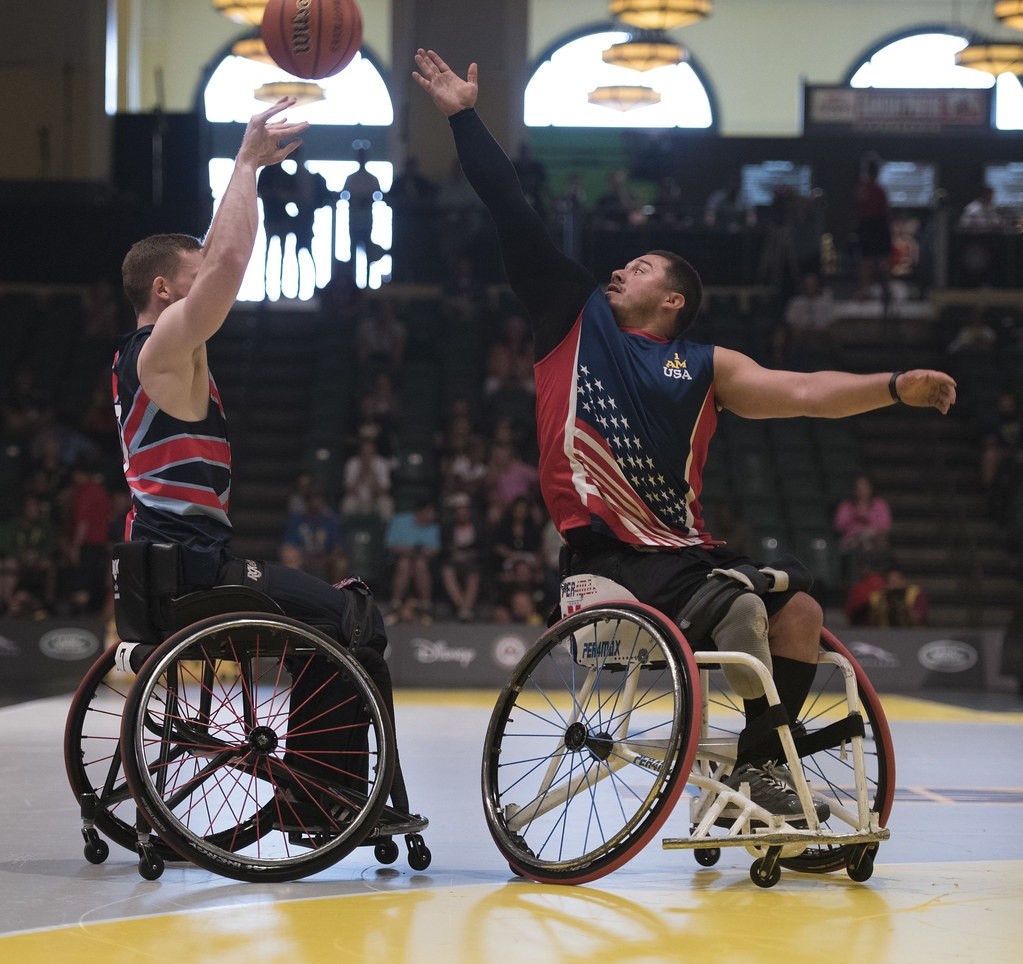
[258,0,363,81]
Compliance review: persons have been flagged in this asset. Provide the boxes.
[112,95,427,835]
[0,139,1023,631]
[411,50,957,823]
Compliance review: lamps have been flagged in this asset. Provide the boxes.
[600,32,690,73]
[590,85,661,106]
[230,36,278,71]
[989,0,1023,30]
[607,2,711,30]
[251,78,326,104]
[954,35,1022,82]
[210,1,267,27]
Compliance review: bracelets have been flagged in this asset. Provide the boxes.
[889,372,904,404]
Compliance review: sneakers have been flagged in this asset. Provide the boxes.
[272,785,338,833]
[319,793,357,824]
[710,758,830,828]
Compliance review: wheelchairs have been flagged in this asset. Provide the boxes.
[479,544,895,889]
[63,539,431,883]
